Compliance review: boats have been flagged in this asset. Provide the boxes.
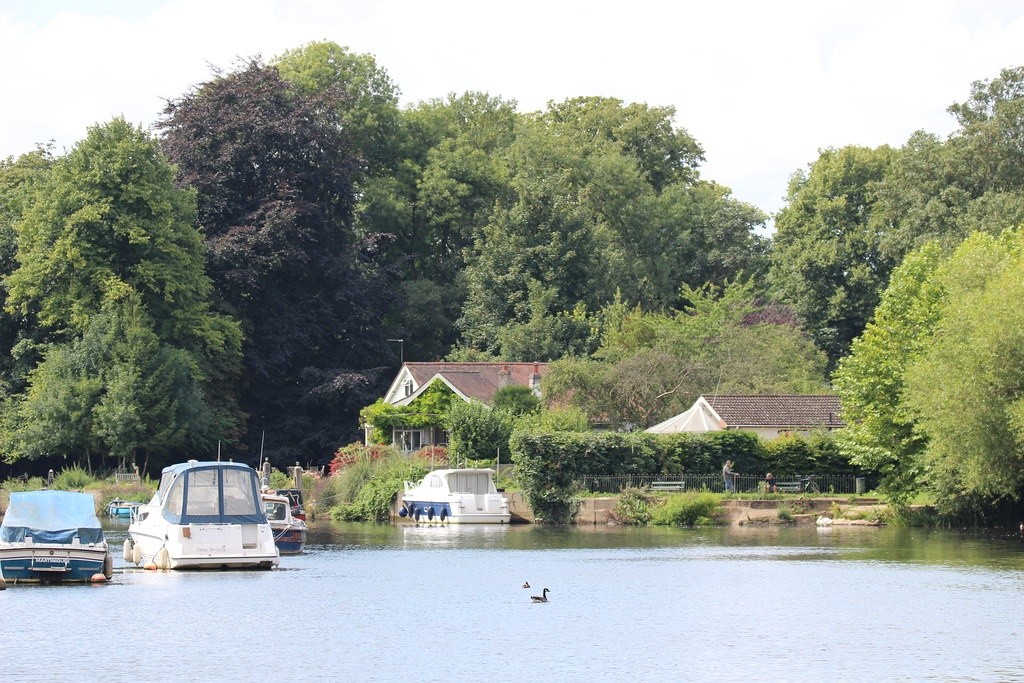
[0,489,114,584]
[107,497,144,518]
[129,457,282,569]
[260,492,306,556]
[399,468,513,524]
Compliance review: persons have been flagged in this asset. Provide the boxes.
[766,474,777,493]
[723,460,740,492]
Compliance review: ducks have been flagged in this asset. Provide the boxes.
[522,582,531,588]
[531,588,551,603]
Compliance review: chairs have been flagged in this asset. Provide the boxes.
[214,496,253,516]
